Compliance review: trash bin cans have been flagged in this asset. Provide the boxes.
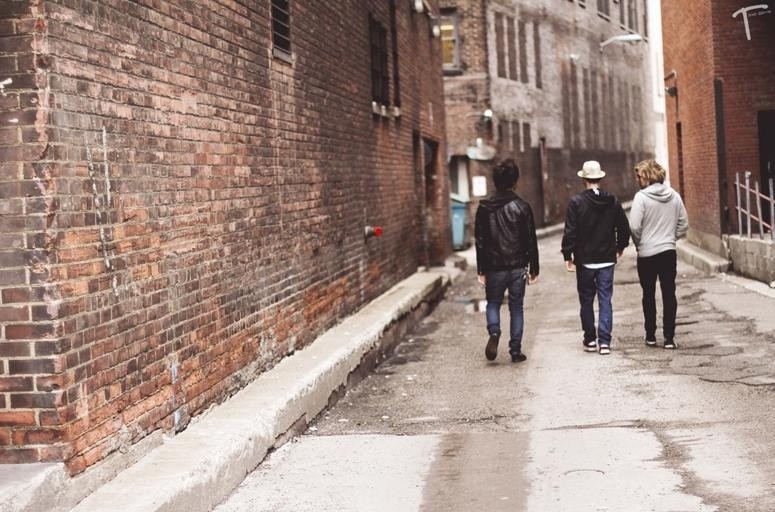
[449,193,472,251]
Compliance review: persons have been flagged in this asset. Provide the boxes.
[628,158,689,349]
[472,159,542,362]
[562,160,632,353]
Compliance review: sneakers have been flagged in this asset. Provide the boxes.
[599,343,611,355]
[512,355,527,362]
[485,332,499,361]
[664,337,676,349]
[583,340,596,352]
[646,336,656,345]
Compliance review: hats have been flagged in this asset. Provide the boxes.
[577,160,606,180]
